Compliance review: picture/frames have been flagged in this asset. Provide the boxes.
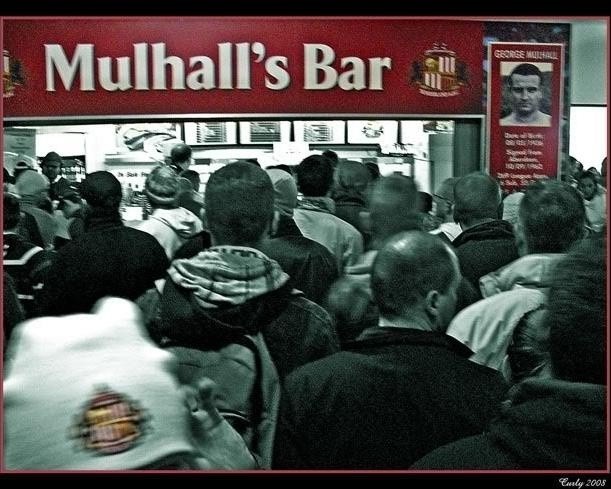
[485,42,565,193]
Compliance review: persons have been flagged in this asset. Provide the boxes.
[499,62,553,128]
[1,233,201,469]
[272,230,507,471]
[381,155,607,299]
[135,163,342,360]
[409,237,607,470]
[324,175,476,338]
[449,179,588,375]
[3,143,212,322]
[264,149,381,296]
[162,378,259,469]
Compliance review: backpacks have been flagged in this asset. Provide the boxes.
[161,332,281,470]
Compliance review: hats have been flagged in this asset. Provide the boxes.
[266,168,298,217]
[144,165,182,205]
[0,295,195,474]
[15,170,52,197]
